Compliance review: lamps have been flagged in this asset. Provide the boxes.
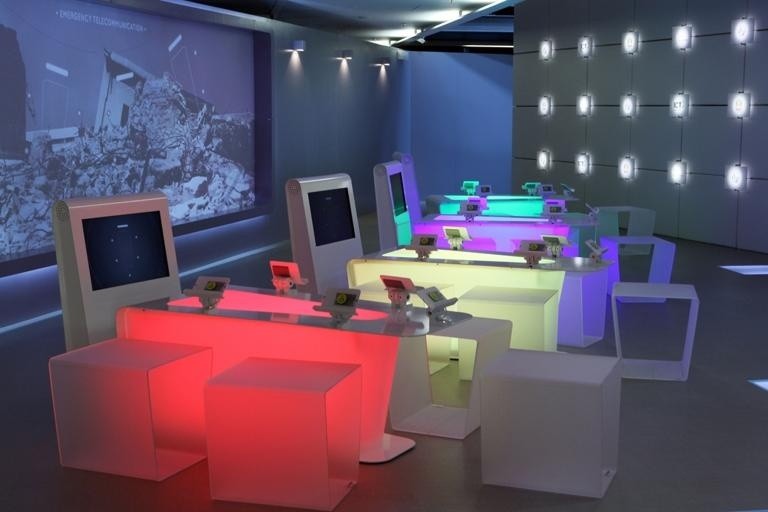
[667,160,687,184]
[574,152,592,174]
[537,94,552,116]
[619,155,638,181]
[536,148,552,171]
[671,24,692,50]
[726,92,750,121]
[621,31,638,54]
[371,57,391,68]
[541,39,556,60]
[333,50,353,60]
[576,94,592,116]
[669,93,689,118]
[577,34,595,58]
[620,95,640,119]
[732,17,756,45]
[724,164,748,191]
[276,38,305,55]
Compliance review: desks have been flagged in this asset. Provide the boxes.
[115,288,472,465]
[413,213,601,263]
[425,194,548,221]
[346,246,616,349]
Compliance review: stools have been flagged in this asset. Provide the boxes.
[480,349,623,499]
[457,286,559,383]
[597,234,678,304]
[611,281,701,384]
[388,316,512,441]
[590,206,657,254]
[205,359,363,510]
[47,336,215,480]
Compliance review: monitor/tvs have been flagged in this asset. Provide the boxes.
[307,187,356,246]
[81,210,171,292]
[389,172,407,217]
